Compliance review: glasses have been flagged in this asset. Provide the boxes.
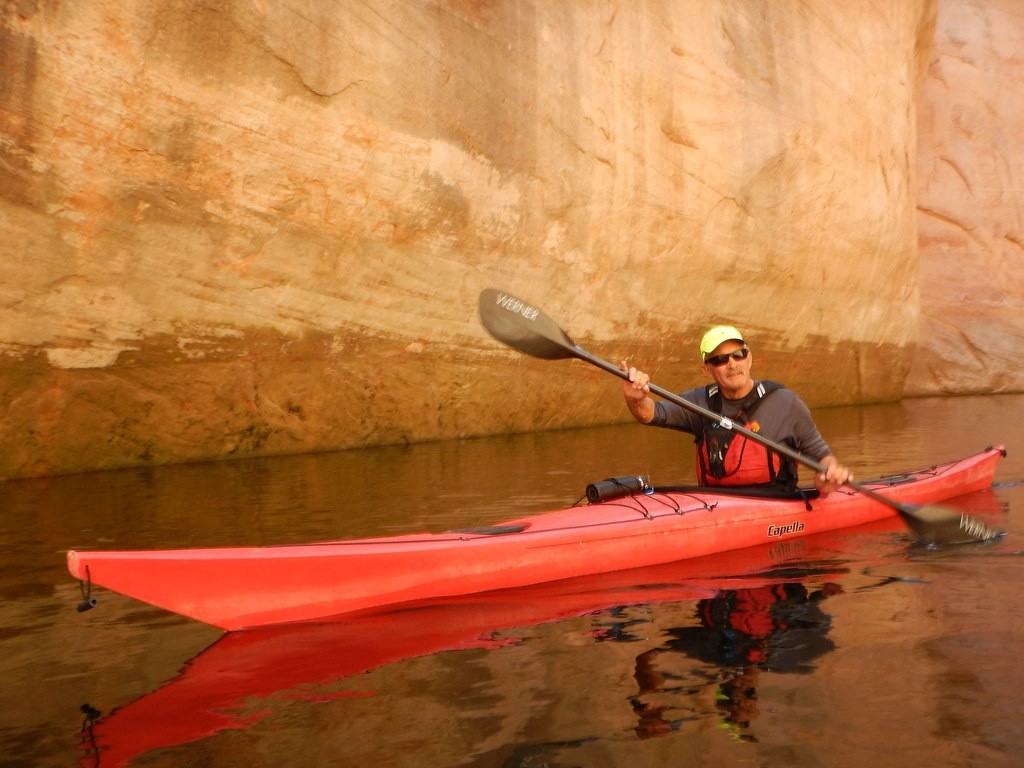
[705,349,748,366]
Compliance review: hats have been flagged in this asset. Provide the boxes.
[700,325,745,361]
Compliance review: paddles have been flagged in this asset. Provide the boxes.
[478,286,994,544]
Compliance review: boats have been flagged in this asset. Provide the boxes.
[66,440,1008,630]
[81,480,1008,767]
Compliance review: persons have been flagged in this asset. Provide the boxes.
[620,326,854,494]
